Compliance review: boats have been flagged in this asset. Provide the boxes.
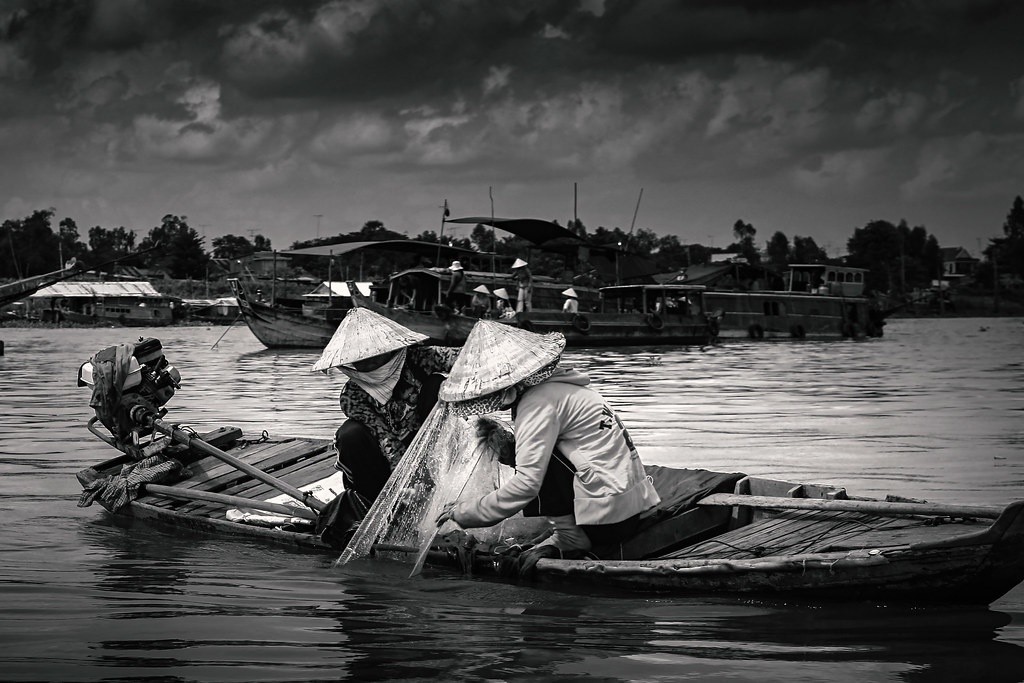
[436,274,722,347]
[237,240,497,350]
[92,294,186,329]
[346,200,635,343]
[74,335,1024,610]
[704,261,890,341]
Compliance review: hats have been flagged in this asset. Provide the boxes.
[493,288,509,299]
[474,285,490,294]
[449,261,463,271]
[511,258,528,268]
[562,288,578,298]
[438,317,565,401]
[310,308,430,372]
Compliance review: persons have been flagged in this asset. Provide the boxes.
[309,307,466,534]
[434,319,661,543]
[505,258,532,312]
[562,287,579,313]
[445,261,466,315]
[493,287,511,310]
[471,285,491,319]
